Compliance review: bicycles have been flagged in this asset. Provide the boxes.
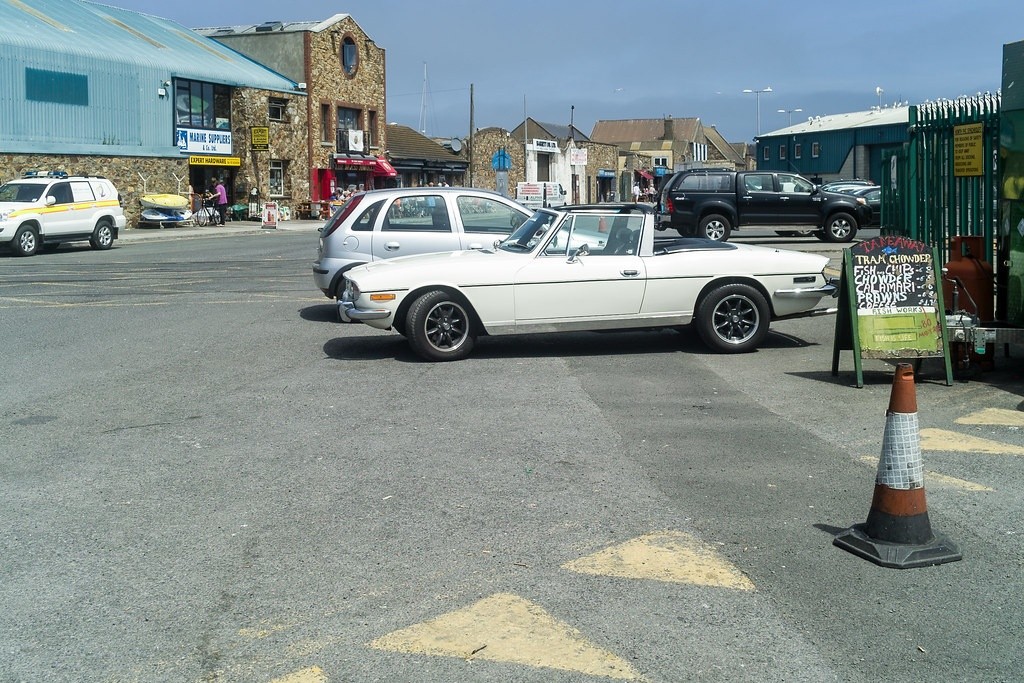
[197,198,222,227]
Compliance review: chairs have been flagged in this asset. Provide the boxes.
[613,228,640,255]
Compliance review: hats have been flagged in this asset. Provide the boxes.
[330,196,336,200]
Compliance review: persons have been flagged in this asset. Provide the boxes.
[203,177,227,227]
[387,199,402,218]
[642,184,656,204]
[418,181,450,217]
[634,184,639,204]
[329,184,365,213]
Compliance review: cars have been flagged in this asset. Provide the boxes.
[746,175,998,239]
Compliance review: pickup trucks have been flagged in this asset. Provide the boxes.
[652,167,875,243]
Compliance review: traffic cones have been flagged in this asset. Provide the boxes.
[833,363,963,569]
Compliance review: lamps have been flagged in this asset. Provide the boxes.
[164,81,171,86]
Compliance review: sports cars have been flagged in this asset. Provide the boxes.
[336,199,841,363]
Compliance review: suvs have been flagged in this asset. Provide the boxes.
[312,186,610,302]
[0,170,128,258]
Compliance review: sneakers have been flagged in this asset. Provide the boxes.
[216,223,225,227]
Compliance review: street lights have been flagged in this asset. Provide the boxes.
[742,87,773,136]
[778,108,803,127]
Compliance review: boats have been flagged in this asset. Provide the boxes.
[138,193,191,210]
[140,206,193,222]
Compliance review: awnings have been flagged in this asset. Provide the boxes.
[635,169,653,179]
[374,159,397,176]
